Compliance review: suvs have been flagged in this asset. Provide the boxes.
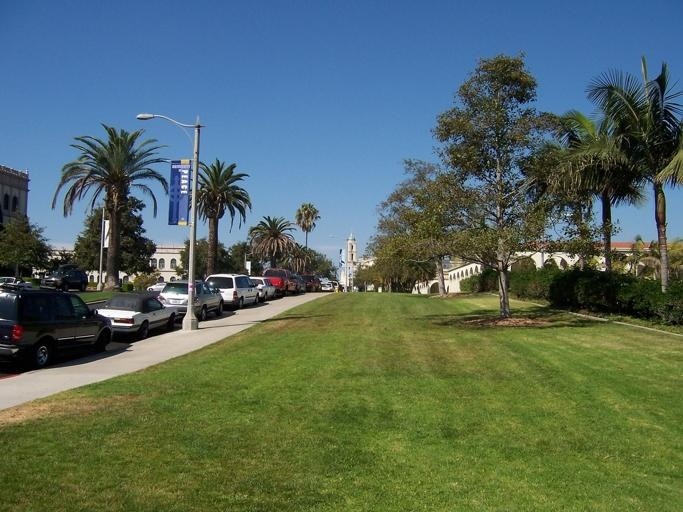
[0,283,113,370]
[39,264,89,292]
[202,274,260,310]
[156,279,225,322]
[261,267,298,297]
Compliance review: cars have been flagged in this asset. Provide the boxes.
[146,282,166,293]
[0,277,33,289]
[302,275,322,293]
[94,292,179,341]
[291,274,306,294]
[321,280,343,293]
[248,277,276,303]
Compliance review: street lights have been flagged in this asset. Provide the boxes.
[137,112,205,331]
